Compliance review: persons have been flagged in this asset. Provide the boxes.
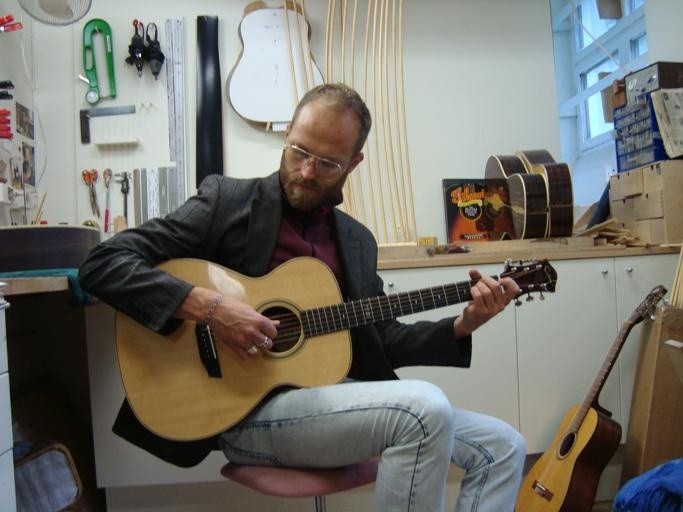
[78,83,527,512]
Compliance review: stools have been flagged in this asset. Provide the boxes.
[220,459,378,511]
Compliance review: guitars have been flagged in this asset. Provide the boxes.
[114,256,557,441]
[515,285,668,512]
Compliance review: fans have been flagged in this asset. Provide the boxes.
[19,0,93,27]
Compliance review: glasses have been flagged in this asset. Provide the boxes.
[282,134,351,182]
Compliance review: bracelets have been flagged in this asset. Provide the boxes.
[204,293,223,324]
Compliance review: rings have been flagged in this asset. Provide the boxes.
[247,344,258,355]
[258,335,270,348]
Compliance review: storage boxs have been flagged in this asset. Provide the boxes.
[617,304,683,491]
[599,61,683,246]
